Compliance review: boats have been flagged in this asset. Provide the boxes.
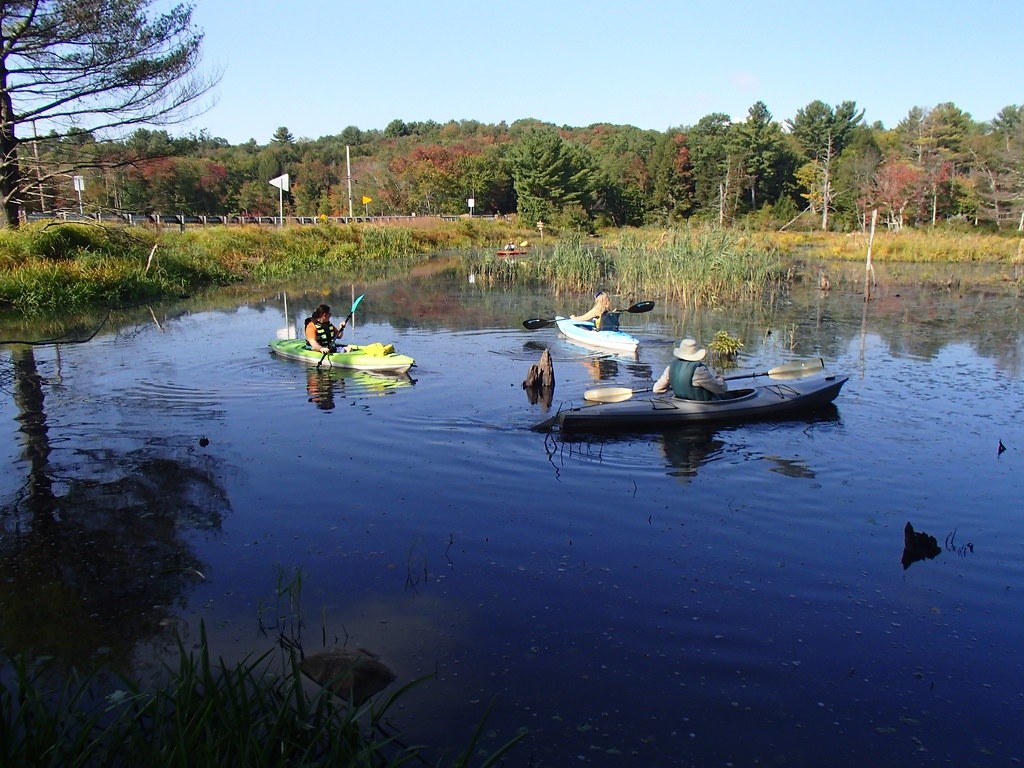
[558,374,849,433]
[497,249,528,255]
[268,339,415,375]
[555,314,640,354]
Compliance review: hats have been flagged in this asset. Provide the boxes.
[594,289,604,298]
[673,339,708,360]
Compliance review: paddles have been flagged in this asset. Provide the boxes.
[316,294,366,370]
[584,358,825,403]
[523,301,656,330]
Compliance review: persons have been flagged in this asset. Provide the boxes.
[305,303,345,354]
[653,339,728,402]
[505,243,515,251]
[570,287,617,331]
